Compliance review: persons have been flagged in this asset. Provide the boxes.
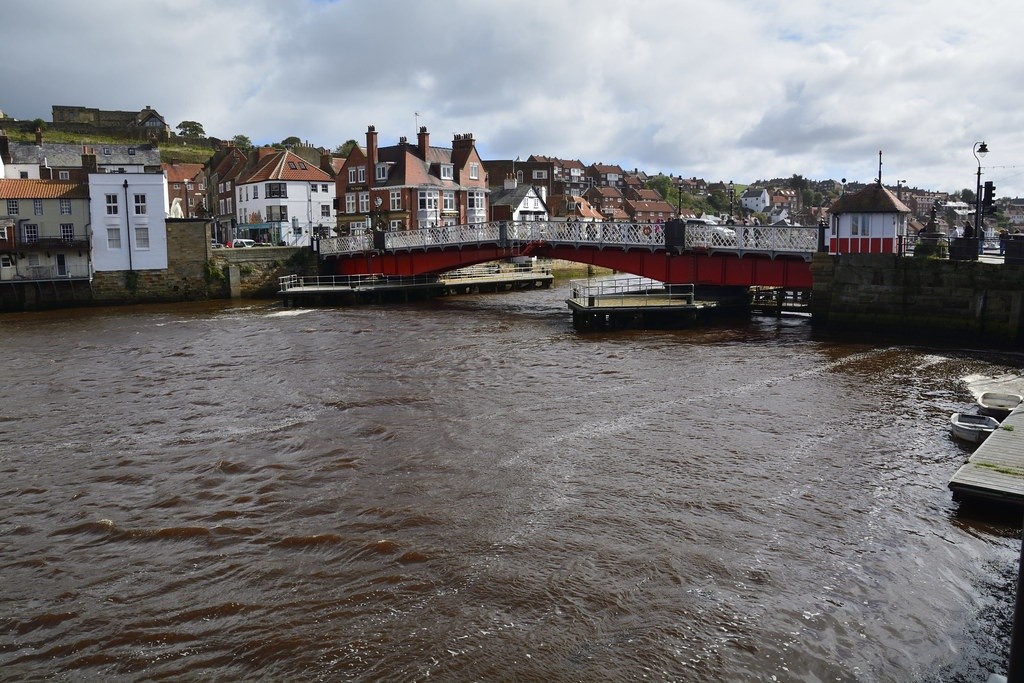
[743,218,760,247]
[950,221,985,253]
[567,216,671,243]
[997,228,1018,254]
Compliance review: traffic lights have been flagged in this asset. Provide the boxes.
[983,181,997,215]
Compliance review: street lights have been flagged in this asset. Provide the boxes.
[676,175,685,220]
[727,181,735,220]
[973,141,990,239]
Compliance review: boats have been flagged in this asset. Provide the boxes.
[976,392,1023,419]
[950,412,1003,445]
